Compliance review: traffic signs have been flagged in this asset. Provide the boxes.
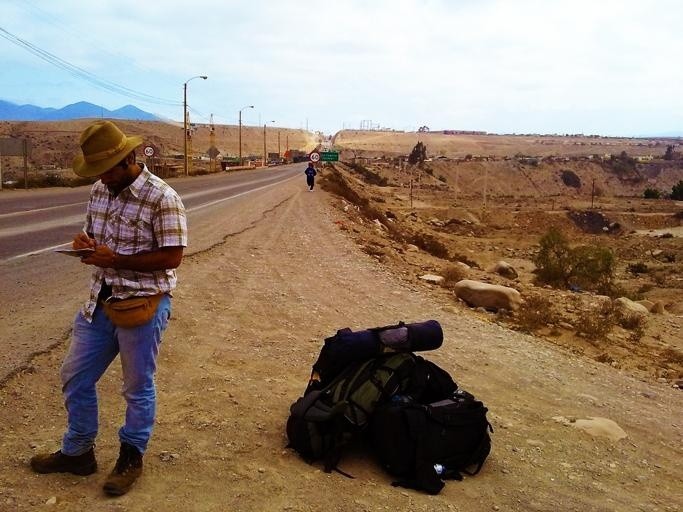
[309,152,320,163]
[319,152,339,161]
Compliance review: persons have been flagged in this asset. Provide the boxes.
[30,119,189,497]
[304,162,316,190]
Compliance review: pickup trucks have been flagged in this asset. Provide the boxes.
[270,159,279,164]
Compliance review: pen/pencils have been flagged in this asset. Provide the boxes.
[82,228,95,249]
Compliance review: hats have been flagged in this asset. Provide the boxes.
[74,120,142,180]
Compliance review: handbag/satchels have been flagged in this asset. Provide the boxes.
[98,293,162,325]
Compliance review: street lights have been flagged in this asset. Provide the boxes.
[286,132,294,152]
[277,128,287,158]
[238,104,256,164]
[263,119,276,167]
[182,75,212,176]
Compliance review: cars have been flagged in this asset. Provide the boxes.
[291,154,309,163]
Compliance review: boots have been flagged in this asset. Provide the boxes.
[104,441,145,496]
[31,449,96,477]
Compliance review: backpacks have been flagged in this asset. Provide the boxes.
[284,319,494,494]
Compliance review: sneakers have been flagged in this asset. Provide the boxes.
[310,186,316,193]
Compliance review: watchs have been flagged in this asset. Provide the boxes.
[112,254,120,271]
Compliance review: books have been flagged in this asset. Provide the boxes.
[52,248,96,257]
